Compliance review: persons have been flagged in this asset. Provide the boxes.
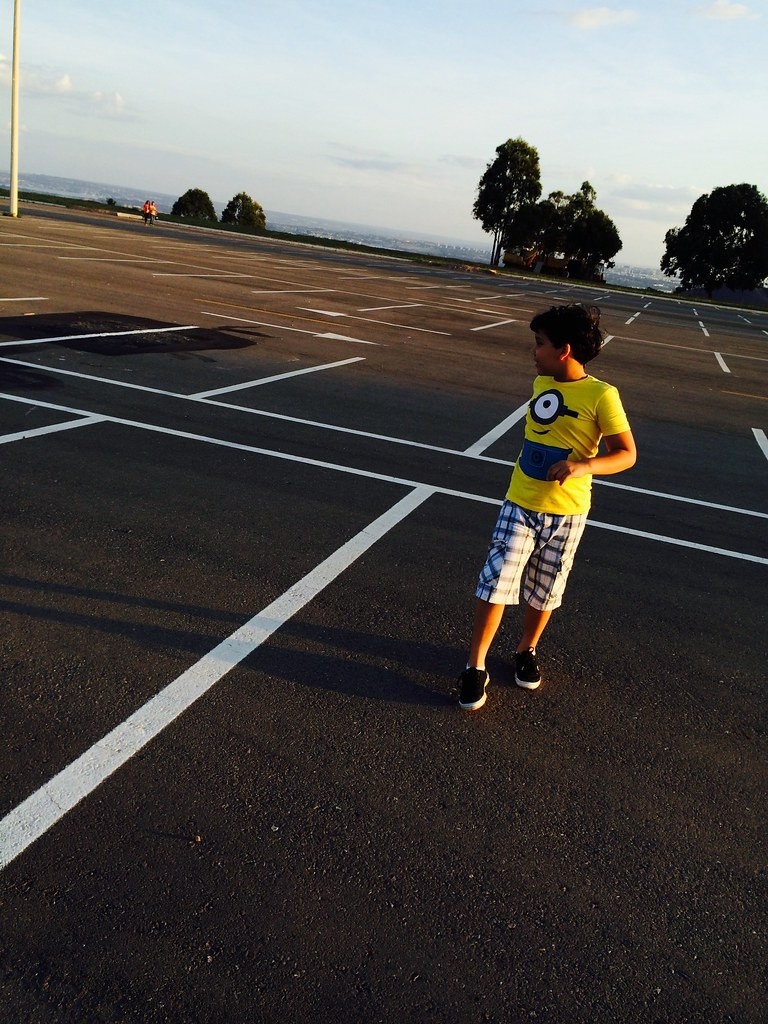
[144,200,150,226]
[148,202,158,226]
[458,304,637,708]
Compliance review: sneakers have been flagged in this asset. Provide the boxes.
[512,651,542,690]
[456,666,490,711]
